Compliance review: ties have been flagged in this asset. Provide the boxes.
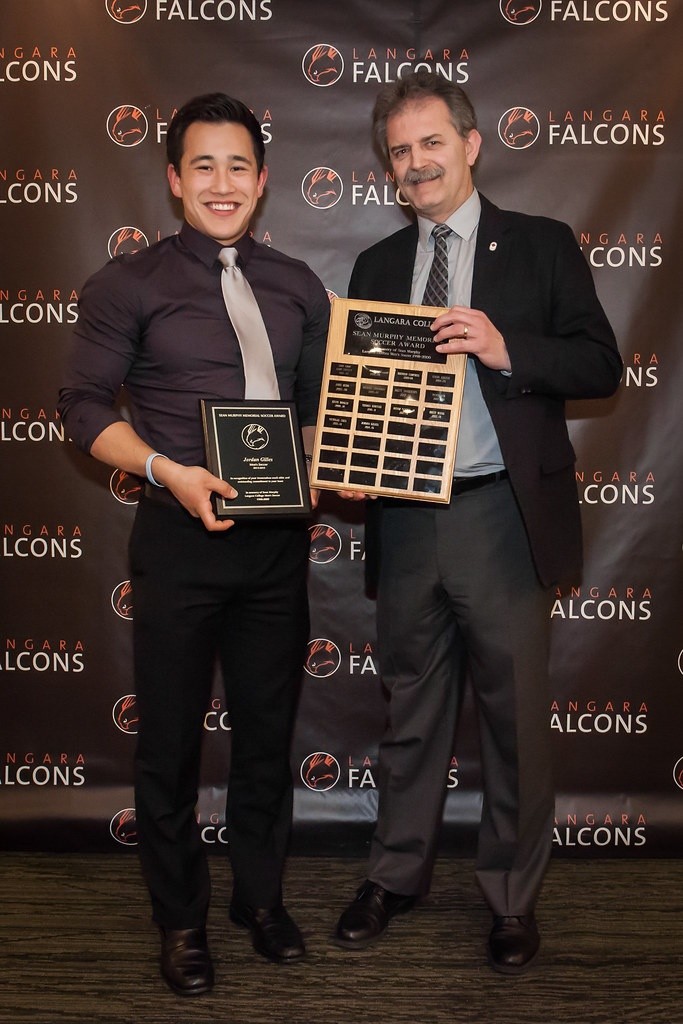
[213,247,281,401]
[420,223,453,307]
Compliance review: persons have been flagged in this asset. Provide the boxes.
[55,93,327,994]
[315,73,625,975]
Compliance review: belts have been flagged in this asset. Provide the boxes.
[451,469,510,496]
[143,480,183,507]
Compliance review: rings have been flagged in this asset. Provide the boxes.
[462,324,470,339]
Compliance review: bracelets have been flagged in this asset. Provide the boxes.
[303,452,313,462]
[146,453,170,489]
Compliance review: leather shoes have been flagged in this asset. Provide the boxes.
[229,887,307,963]
[152,917,212,997]
[488,909,541,975]
[334,878,418,949]
